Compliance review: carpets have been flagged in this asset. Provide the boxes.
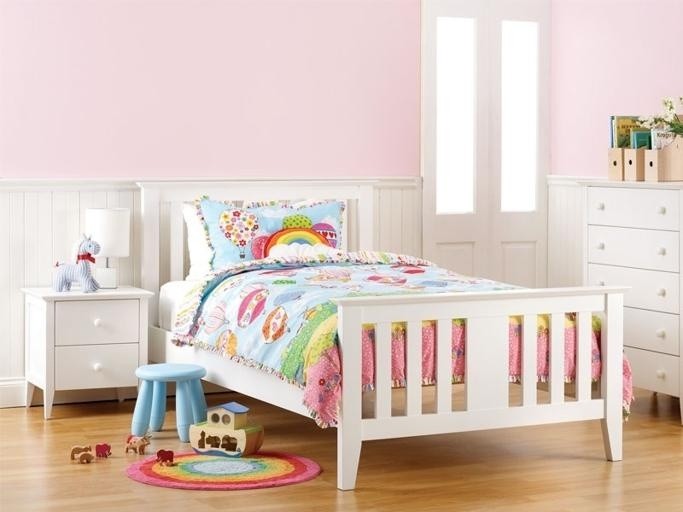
[125,450,321,490]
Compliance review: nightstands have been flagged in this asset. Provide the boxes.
[20,285,155,419]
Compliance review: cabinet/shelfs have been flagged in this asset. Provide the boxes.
[583,181,683,426]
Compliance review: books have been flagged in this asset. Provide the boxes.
[609,115,674,149]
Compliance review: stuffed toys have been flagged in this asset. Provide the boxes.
[53,233,101,294]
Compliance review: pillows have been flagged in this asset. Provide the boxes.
[178,195,345,280]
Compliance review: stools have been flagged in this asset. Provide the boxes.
[131,363,207,442]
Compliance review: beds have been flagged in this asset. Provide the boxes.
[137,182,633,490]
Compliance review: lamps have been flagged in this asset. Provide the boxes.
[85,207,130,288]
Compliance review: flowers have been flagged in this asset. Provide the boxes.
[630,95,683,138]
